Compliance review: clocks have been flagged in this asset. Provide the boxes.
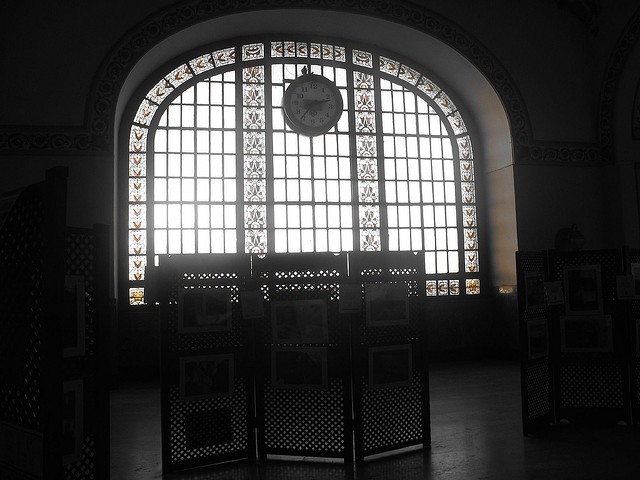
[290,80,337,128]
